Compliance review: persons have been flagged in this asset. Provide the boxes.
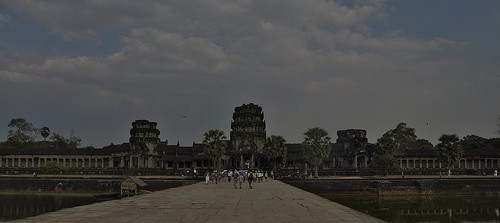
[31,151,495,210]
